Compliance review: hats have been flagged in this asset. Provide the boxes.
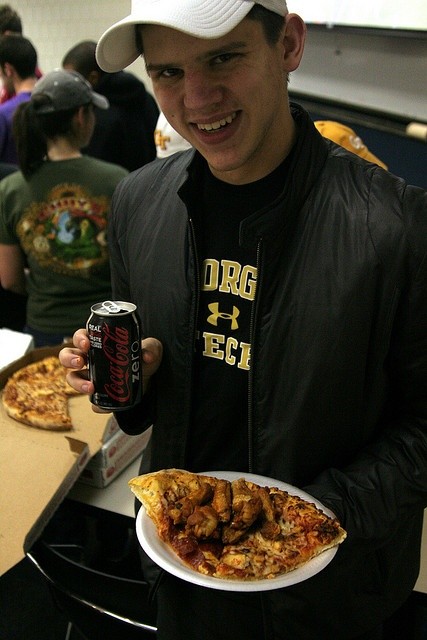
[154,112,192,159]
[31,68,110,115]
[95,0,288,74]
[313,120,389,171]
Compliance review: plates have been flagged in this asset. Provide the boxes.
[136,471,340,592]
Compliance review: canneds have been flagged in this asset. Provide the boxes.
[86,299,143,409]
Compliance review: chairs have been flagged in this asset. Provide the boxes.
[26,500,158,640]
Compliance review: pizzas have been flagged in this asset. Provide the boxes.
[126,468,349,579]
[2,354,85,432]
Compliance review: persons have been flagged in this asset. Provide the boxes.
[0,32,41,166]
[0,5,42,88]
[61,40,160,174]
[0,70,131,347]
[59,0,427,640]
